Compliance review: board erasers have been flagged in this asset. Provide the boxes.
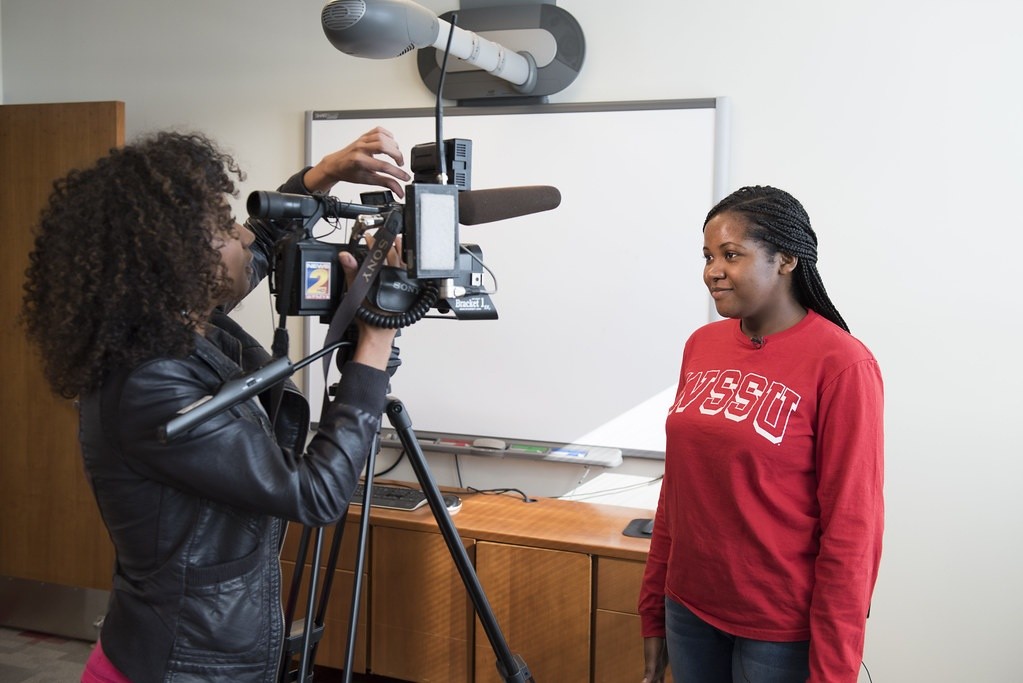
[473,438,507,451]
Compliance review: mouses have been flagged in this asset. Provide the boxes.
[441,493,462,512]
[640,519,655,534]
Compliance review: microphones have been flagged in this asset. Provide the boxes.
[156,354,294,447]
[456,185,561,226]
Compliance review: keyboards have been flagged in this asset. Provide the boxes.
[350,482,428,512]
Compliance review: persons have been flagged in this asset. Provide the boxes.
[23,125,413,683]
[640,187,885,682]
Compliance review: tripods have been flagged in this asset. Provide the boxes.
[275,384,534,683]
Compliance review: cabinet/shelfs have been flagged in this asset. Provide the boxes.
[279,484,682,681]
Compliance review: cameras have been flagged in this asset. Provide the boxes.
[245,137,499,321]
